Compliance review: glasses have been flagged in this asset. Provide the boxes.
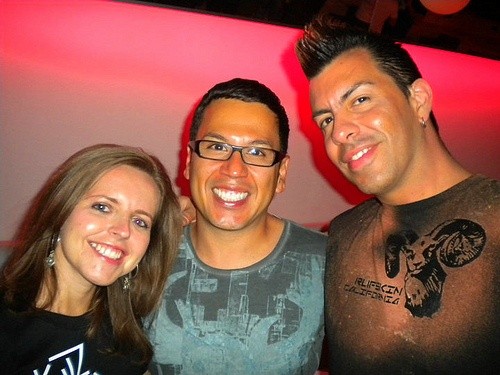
[187,139,287,167]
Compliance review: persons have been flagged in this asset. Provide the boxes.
[174,14,500,375]
[1,144,180,375]
[143,78,328,375]
